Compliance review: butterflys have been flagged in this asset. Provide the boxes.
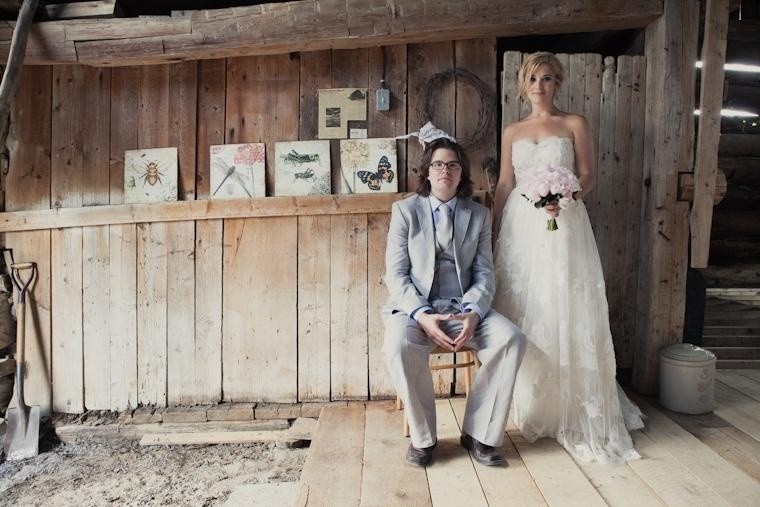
[356,156,395,192]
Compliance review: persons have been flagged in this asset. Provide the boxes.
[489,51,657,472]
[382,136,526,471]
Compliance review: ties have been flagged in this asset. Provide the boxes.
[435,203,454,251]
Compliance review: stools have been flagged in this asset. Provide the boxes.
[392,342,483,438]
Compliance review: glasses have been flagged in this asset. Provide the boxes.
[430,160,461,170]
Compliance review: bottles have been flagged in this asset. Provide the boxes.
[379,80,386,89]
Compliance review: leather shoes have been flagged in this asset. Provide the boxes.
[460,434,502,467]
[405,436,438,468]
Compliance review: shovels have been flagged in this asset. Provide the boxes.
[4,260,42,462]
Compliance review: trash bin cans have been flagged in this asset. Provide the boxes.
[658,341,717,416]
